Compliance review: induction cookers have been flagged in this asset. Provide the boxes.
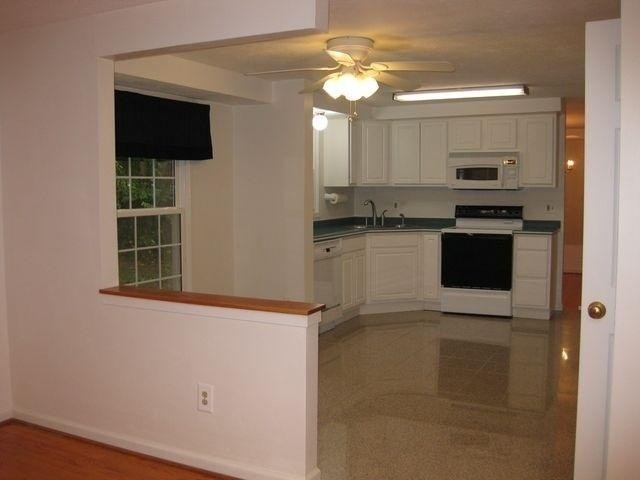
[445,226,515,230]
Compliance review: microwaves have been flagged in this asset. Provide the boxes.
[448,156,519,192]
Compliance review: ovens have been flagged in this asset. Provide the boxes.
[437,337,511,415]
[440,232,514,289]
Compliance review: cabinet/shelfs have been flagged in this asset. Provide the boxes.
[420,232,440,302]
[366,231,420,314]
[516,113,558,188]
[512,231,553,320]
[321,118,360,188]
[358,120,389,187]
[447,115,519,153]
[314,234,366,335]
[388,117,448,188]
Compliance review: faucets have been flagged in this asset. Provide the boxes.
[399,212,404,225]
[364,199,378,227]
[380,209,388,227]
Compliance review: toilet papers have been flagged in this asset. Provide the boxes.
[331,193,348,205]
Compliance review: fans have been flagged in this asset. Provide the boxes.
[246,38,455,125]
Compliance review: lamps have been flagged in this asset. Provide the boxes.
[313,107,328,131]
[564,160,576,177]
[322,69,380,122]
[391,83,529,103]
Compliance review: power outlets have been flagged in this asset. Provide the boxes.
[544,203,556,213]
[393,200,399,211]
[197,382,214,413]
